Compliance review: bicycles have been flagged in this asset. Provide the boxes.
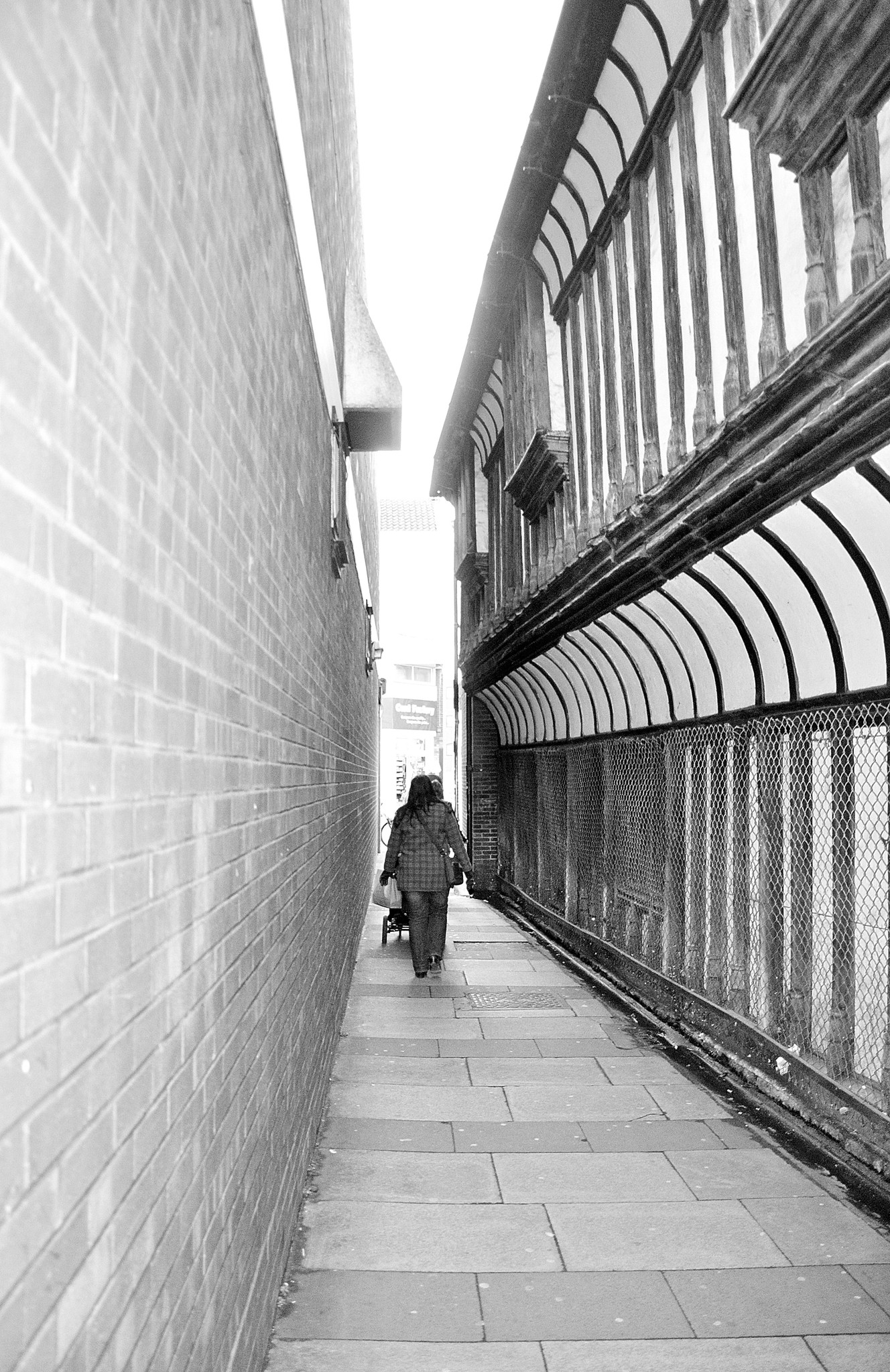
[380,813,394,847]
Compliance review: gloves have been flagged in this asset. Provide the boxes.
[465,871,475,885]
[379,870,395,886]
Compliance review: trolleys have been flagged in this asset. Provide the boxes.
[380,890,409,945]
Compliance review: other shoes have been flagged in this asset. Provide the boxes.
[414,972,428,979]
[430,958,443,974]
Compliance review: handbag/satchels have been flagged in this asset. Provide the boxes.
[443,855,464,887]
[372,869,403,910]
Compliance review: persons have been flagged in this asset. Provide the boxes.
[380,775,477,979]
